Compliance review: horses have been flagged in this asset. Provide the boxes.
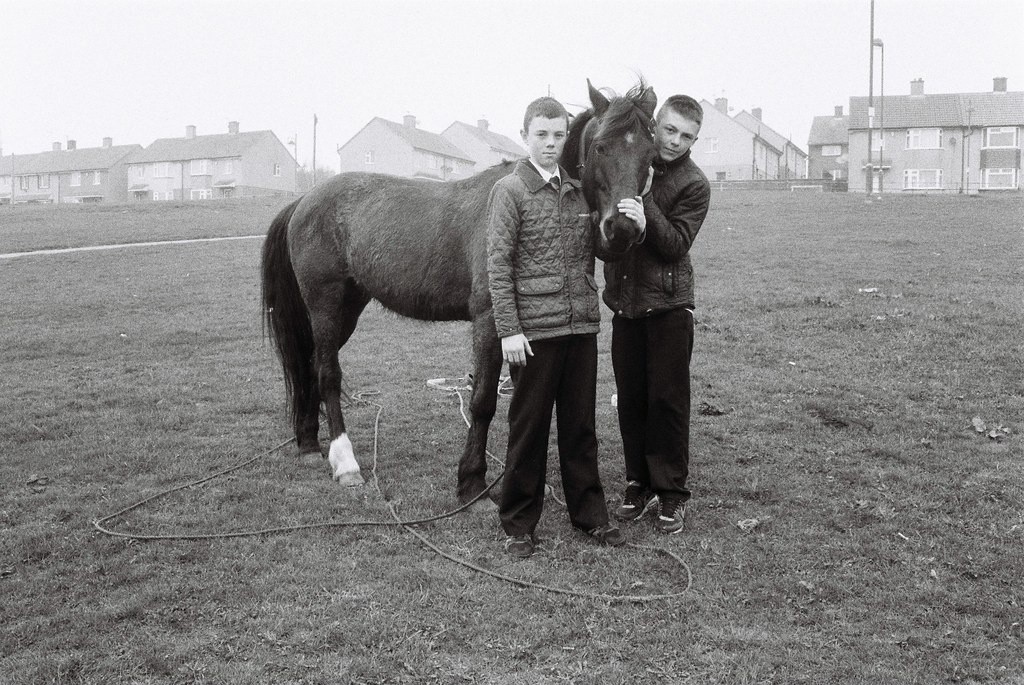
[257,70,660,488]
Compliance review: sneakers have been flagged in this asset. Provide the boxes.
[585,517,626,545]
[505,528,533,558]
[614,481,688,535]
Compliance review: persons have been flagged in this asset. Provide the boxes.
[487,97,646,556]
[603,95,710,532]
